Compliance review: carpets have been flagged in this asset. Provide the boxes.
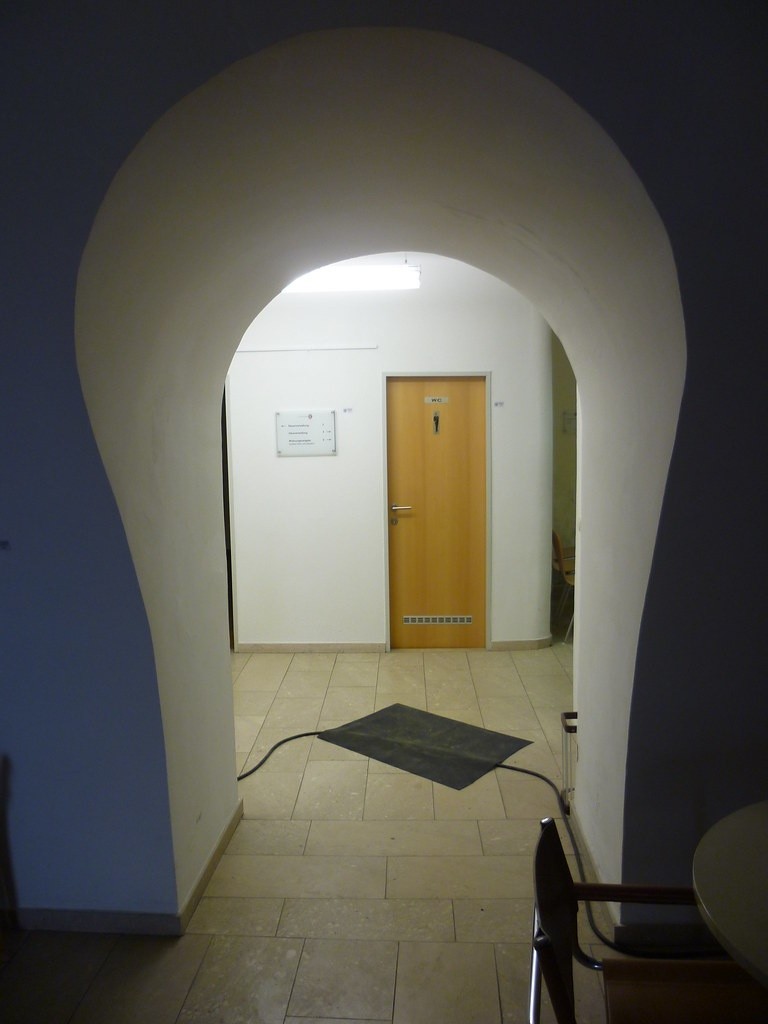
[318,703,534,789]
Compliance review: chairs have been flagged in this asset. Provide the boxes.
[527,817,768,1024]
[552,546,575,590]
[552,557,575,618]
[553,530,576,641]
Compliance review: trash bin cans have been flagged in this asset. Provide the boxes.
[557,712,577,815]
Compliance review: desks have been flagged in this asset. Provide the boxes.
[692,800,768,989]
